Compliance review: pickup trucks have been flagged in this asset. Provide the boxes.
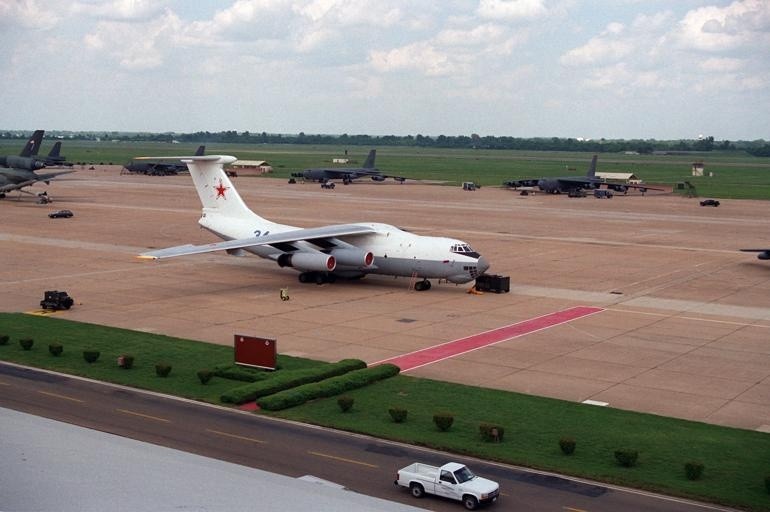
[394,461,500,510]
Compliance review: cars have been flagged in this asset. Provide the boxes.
[700,199,721,207]
[47,209,74,219]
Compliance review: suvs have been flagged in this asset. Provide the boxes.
[39,290,73,311]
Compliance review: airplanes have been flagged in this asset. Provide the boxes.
[740,248,770,261]
[0,130,78,199]
[502,154,665,198]
